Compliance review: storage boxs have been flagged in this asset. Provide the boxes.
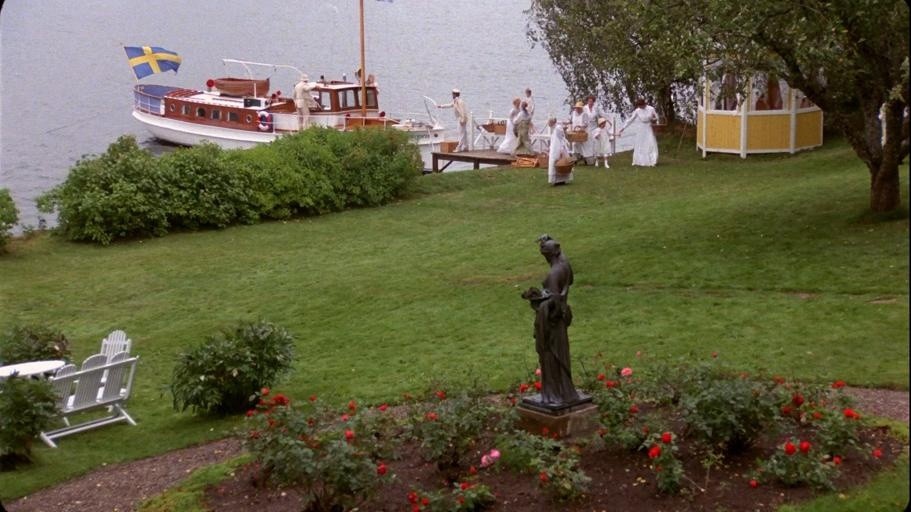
[440,141,460,153]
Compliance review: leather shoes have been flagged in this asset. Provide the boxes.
[594,162,610,168]
[452,148,461,152]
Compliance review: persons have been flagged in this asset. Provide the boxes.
[497,97,535,155]
[583,95,613,144]
[292,74,320,132]
[593,117,622,169]
[521,233,582,407]
[548,117,574,186]
[524,89,535,117]
[365,74,381,95]
[511,101,535,157]
[437,88,471,153]
[619,99,660,167]
[569,101,595,165]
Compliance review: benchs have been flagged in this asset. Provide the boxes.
[30,351,139,451]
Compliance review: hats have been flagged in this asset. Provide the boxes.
[452,89,460,93]
[573,102,584,108]
[301,74,309,81]
[598,118,606,125]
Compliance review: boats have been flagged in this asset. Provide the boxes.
[129,0,447,171]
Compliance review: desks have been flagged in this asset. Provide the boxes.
[531,135,552,153]
[0,360,65,381]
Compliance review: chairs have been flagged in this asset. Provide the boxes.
[72,329,132,385]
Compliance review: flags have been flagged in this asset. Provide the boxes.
[124,46,183,79]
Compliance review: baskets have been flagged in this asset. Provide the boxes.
[538,152,549,168]
[554,151,576,173]
[483,119,506,135]
[566,126,587,142]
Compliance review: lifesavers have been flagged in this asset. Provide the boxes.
[256,111,272,131]
